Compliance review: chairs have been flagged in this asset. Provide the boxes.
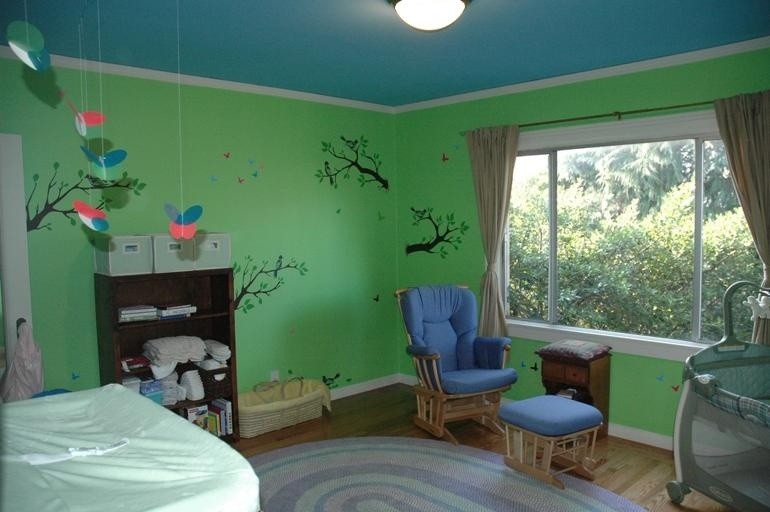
[395,284,518,439]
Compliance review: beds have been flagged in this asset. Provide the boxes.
[0,383,260,512]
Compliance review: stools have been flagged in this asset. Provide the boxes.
[498,393,604,489]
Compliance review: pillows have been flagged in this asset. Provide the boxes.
[534,340,613,361]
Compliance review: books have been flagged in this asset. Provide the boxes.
[117,300,198,327]
[181,396,234,440]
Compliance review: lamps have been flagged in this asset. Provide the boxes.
[387,0,473,33]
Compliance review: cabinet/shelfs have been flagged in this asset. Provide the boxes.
[94,268,240,445]
[541,353,612,442]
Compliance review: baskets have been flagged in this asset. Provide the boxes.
[238,376,323,439]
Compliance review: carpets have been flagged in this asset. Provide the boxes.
[244,435,652,512]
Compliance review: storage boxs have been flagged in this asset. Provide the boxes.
[95,233,229,277]
